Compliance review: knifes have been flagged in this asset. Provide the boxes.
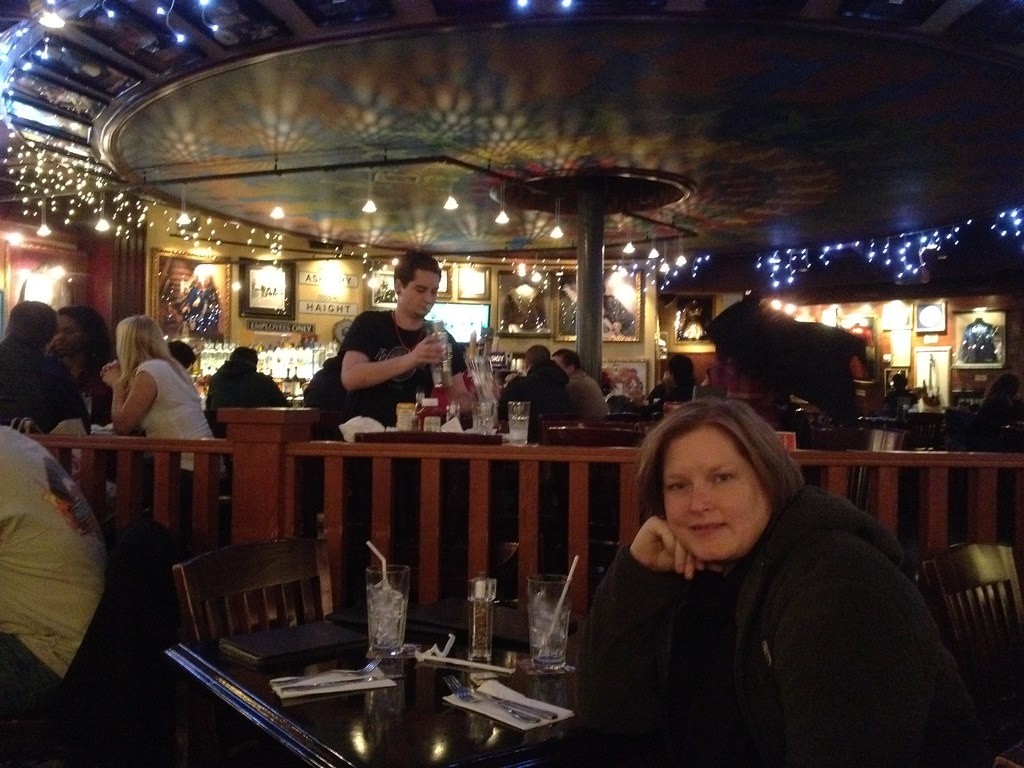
[281,674,409,692]
[464,686,558,720]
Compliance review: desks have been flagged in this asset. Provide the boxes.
[164,596,621,768]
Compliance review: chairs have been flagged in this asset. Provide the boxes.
[205,408,232,477]
[352,407,1024,756]
[175,521,334,647]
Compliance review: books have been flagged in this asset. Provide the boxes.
[219,621,369,672]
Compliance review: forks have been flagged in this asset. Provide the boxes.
[442,675,541,723]
[269,656,384,687]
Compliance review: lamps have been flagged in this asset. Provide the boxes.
[37,154,687,276]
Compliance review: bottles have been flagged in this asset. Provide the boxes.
[465,571,498,663]
[396,392,441,431]
[445,401,461,424]
[181,337,338,398]
[422,320,447,342]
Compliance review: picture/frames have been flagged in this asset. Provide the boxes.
[950,308,1007,369]
[913,298,946,332]
[551,268,643,343]
[436,266,452,299]
[150,247,231,344]
[458,265,489,300]
[491,268,553,338]
[238,255,296,322]
[372,270,398,308]
[914,346,951,414]
[664,291,723,352]
[602,359,649,398]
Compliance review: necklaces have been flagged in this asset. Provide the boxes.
[393,309,425,355]
[205,348,297,470]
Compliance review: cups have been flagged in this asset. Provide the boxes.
[525,574,575,671]
[470,401,494,433]
[365,564,412,655]
[429,342,454,388]
[507,401,531,446]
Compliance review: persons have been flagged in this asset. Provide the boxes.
[301,249,518,568]
[975,373,1024,451]
[696,291,880,442]
[498,345,610,442]
[0,304,227,540]
[569,397,1000,768]
[0,423,106,767]
[883,372,928,421]
[640,353,723,419]
